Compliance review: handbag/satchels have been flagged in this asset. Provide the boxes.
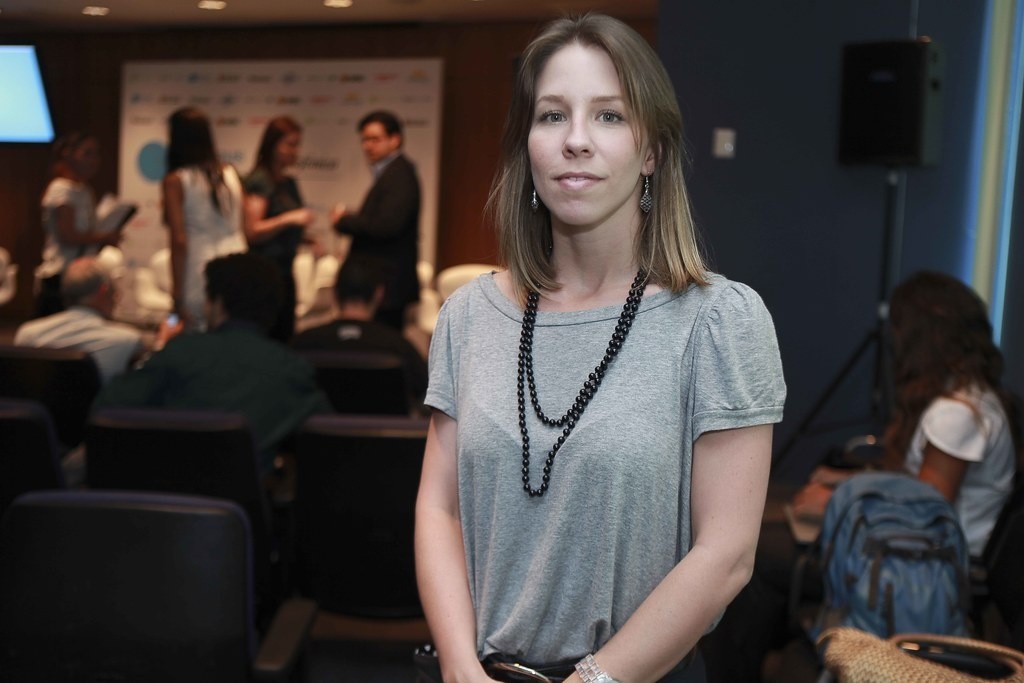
[813,626,1024,682]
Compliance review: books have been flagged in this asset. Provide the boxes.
[91,191,134,239]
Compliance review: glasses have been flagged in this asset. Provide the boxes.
[359,135,381,143]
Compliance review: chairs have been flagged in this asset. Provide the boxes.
[0,344,102,448]
[817,631,1024,683]
[0,495,317,683]
[86,406,287,621]
[296,416,461,659]
[789,391,1024,639]
[0,400,70,496]
[284,352,426,416]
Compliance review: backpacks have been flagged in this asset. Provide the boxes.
[816,469,997,640]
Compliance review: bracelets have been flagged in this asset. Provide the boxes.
[574,653,620,683]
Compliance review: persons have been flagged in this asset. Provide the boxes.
[763,275,1015,683]
[162,106,250,331]
[96,249,336,460]
[16,252,155,391]
[244,117,317,277]
[33,134,121,317]
[327,106,423,335]
[412,9,788,683]
[295,262,429,420]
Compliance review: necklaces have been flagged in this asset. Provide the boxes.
[517,267,649,498]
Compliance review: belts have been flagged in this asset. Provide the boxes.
[413,643,698,683]
